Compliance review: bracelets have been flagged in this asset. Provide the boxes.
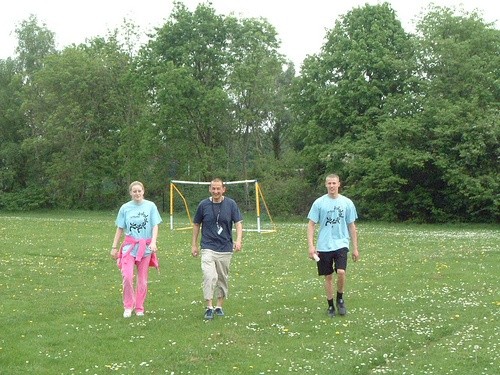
[111,247,117,249]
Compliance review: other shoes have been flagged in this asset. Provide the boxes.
[123,309,146,319]
[327,298,345,317]
[204,307,224,318]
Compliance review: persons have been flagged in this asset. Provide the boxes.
[191,178,244,319]
[307,173,359,317]
[111,181,163,318]
